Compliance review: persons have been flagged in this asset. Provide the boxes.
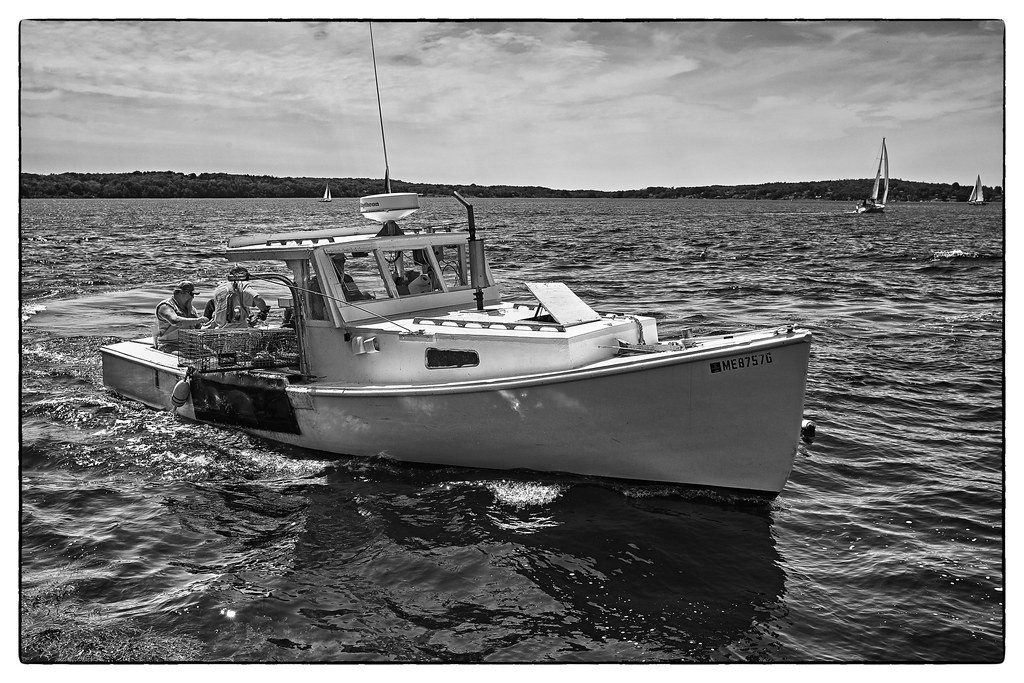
[310,254,364,320]
[214,265,271,328]
[155,281,209,356]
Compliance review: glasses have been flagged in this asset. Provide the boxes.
[185,290,194,296]
[335,260,345,263]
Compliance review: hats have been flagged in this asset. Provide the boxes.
[175,281,200,294]
[330,253,348,259]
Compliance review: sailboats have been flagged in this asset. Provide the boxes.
[967,175,985,206]
[852,137,889,213]
[319,181,333,202]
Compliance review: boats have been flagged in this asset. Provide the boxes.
[356,21,419,223]
[97,188,816,514]
